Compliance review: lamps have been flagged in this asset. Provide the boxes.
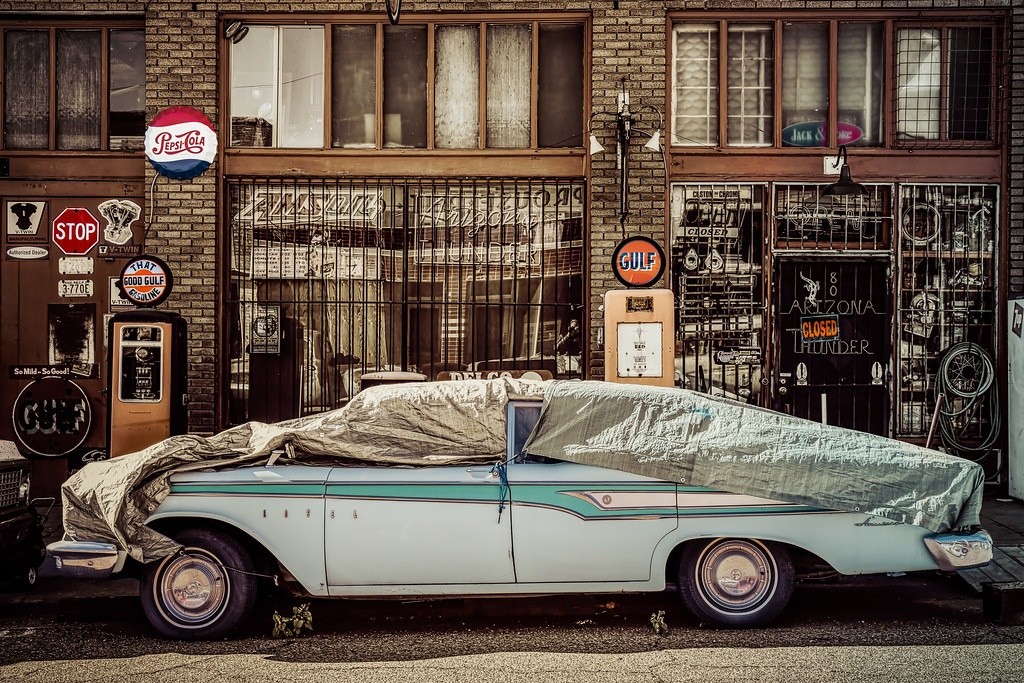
[823,145,869,194]
[587,104,663,156]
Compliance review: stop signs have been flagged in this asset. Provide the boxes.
[52,207,99,255]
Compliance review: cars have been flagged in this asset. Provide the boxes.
[45,375,993,643]
